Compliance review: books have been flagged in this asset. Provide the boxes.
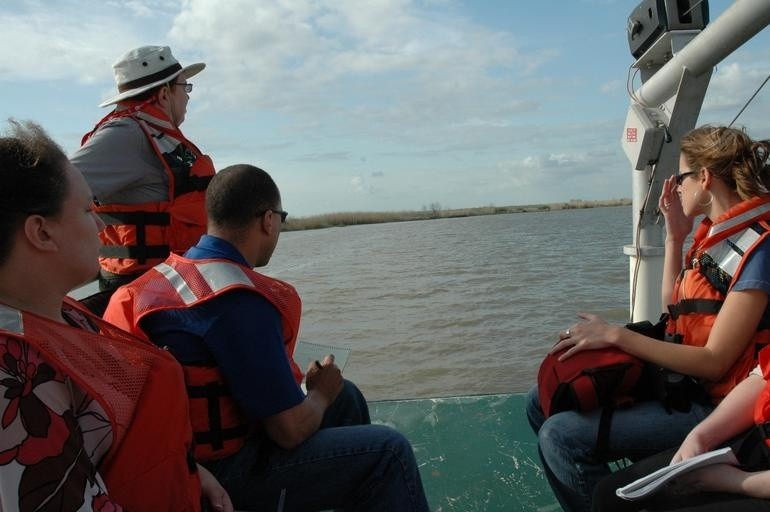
[616,447,740,501]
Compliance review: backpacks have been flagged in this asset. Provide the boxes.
[537,318,658,419]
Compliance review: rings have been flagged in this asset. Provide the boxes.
[565,328,570,335]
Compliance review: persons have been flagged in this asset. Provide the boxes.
[592,344,770,512]
[69,44,217,315]
[524,123,770,509]
[0,112,238,512]
[101,162,429,510]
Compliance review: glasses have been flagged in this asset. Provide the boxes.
[271,210,288,223]
[170,83,192,93]
[674,171,695,185]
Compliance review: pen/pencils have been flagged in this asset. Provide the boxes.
[315,360,323,369]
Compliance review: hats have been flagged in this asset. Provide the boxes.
[97,45,207,109]
